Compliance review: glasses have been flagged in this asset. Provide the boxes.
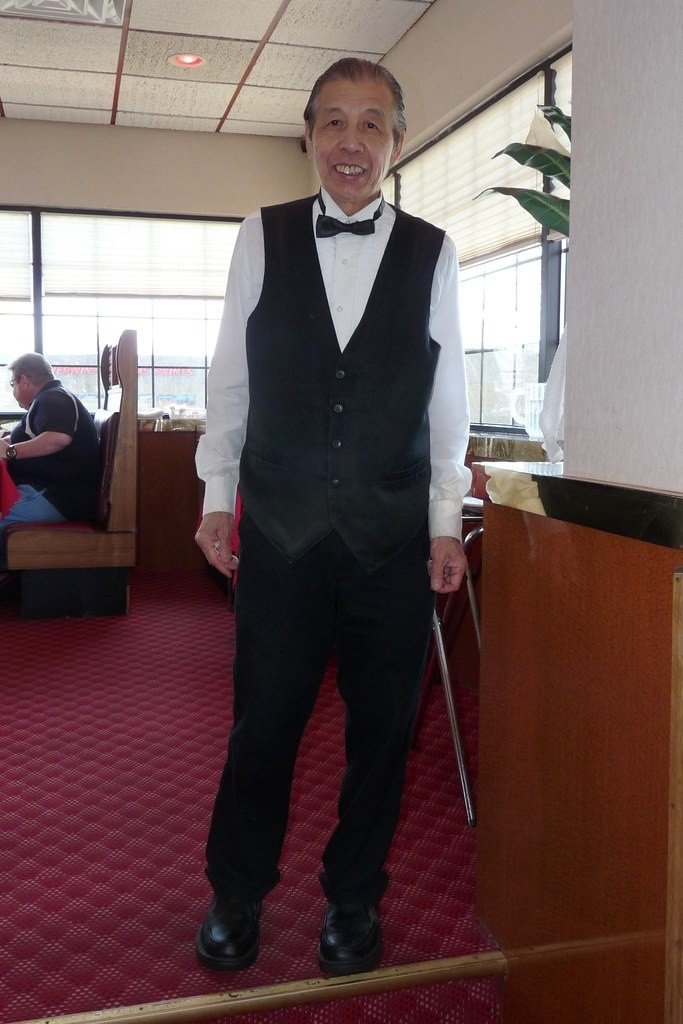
[9,375,30,388]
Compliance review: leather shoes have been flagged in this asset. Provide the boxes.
[318,899,384,976]
[196,893,262,971]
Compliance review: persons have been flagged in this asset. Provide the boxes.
[0,353,99,531]
[194,59,467,980]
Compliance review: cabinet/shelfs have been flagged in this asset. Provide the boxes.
[135,430,208,574]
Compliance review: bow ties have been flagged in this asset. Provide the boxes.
[316,186,385,238]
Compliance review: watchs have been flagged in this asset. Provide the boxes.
[6,444,17,460]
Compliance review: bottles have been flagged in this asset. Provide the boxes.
[162,413,171,431]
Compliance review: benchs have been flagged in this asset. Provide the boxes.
[0,329,139,617]
